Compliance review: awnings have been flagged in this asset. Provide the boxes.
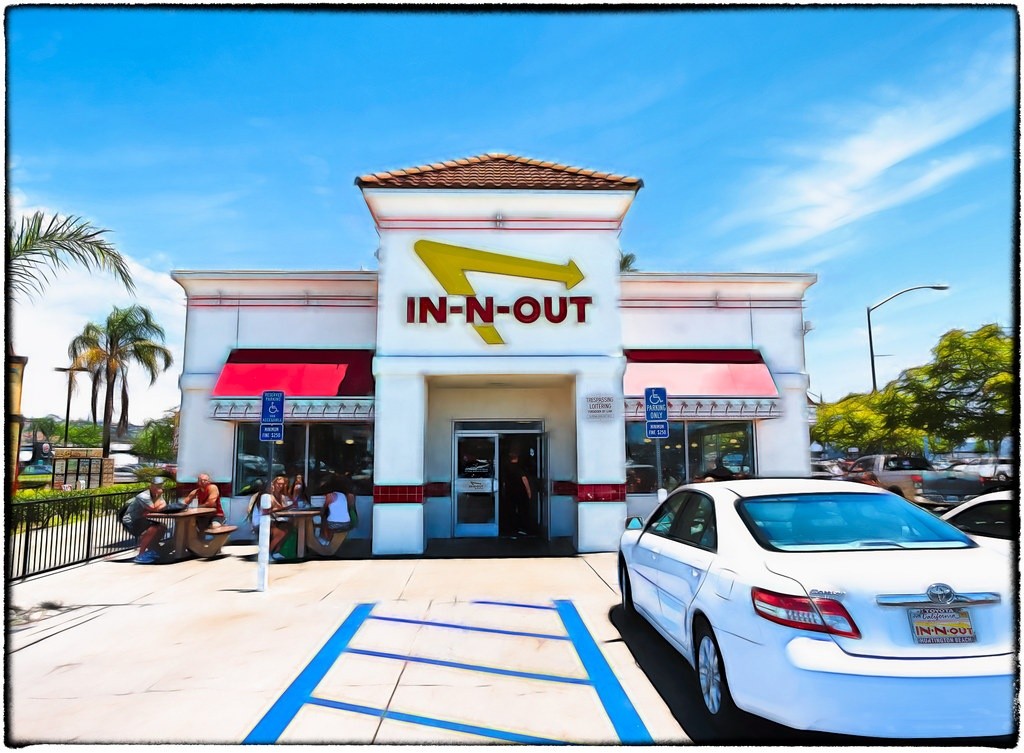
[207,349,376,423]
[623,348,785,421]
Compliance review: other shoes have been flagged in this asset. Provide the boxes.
[317,536,330,546]
[272,552,285,559]
[510,536,518,540]
[518,529,527,536]
[269,554,275,562]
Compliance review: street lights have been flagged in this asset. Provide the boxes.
[866,284,950,394]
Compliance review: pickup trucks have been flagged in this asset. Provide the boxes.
[843,453,987,506]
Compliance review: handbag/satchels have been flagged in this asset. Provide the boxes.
[161,502,188,513]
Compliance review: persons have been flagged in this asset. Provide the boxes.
[122,477,171,564]
[184,473,226,554]
[251,474,352,563]
[502,450,532,540]
[704,457,732,481]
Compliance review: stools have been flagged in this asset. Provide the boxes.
[204,526,238,557]
[305,517,353,556]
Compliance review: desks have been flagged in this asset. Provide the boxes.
[144,508,216,558]
[275,508,323,557]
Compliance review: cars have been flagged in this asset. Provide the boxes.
[811,461,843,478]
[617,479,1016,743]
[936,489,1017,560]
[934,456,1012,480]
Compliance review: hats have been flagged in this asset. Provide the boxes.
[151,477,164,484]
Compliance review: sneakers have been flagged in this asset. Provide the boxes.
[134,555,154,563]
[145,549,161,558]
